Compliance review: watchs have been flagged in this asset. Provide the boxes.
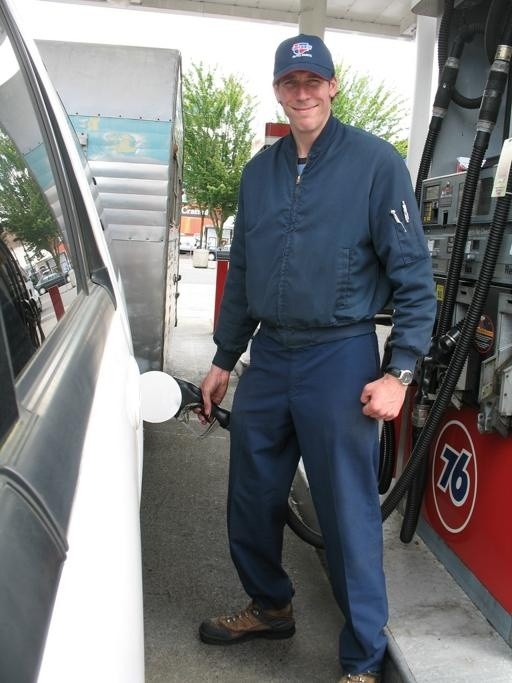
[385,366,415,386]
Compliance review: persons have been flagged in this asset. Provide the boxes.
[189,32,438,683]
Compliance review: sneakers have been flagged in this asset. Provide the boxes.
[199,600,295,645]
[337,665,381,683]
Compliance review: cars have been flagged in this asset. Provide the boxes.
[0,0,182,682]
[179,224,235,260]
[17,265,72,313]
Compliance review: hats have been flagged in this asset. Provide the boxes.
[273,34,335,84]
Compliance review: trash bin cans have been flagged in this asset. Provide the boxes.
[193,248,209,268]
